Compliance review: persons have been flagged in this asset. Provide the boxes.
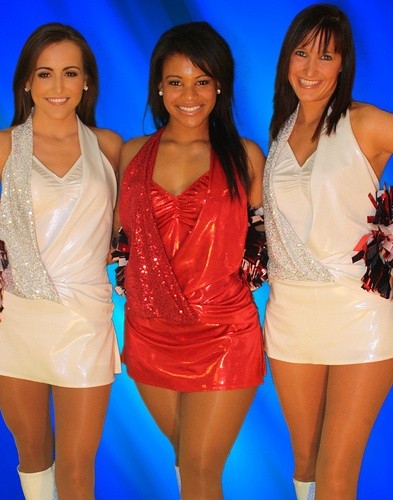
[106,21,268,500]
[263,0,391,500]
[2,18,121,496]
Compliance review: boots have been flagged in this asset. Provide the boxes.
[293,477,316,500]
[17,461,58,500]
